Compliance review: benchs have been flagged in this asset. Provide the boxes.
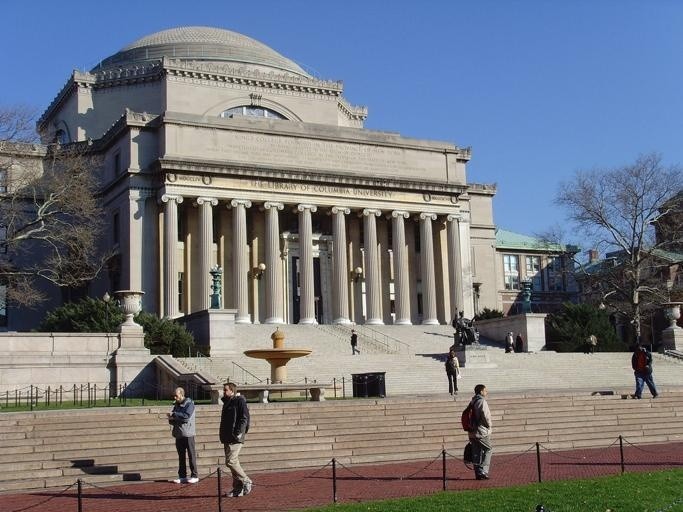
[202,380,333,406]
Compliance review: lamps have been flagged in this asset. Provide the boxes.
[258,263,266,280]
[355,267,363,282]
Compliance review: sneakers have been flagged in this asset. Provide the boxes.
[633,393,658,398]
[451,392,458,395]
[173,477,199,483]
[477,474,490,480]
[225,480,252,497]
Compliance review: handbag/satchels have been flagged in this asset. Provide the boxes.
[463,441,484,465]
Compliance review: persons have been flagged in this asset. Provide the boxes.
[445,351,460,395]
[220,383,252,498]
[505,332,523,353]
[456,311,476,345]
[632,343,658,399]
[351,329,360,354]
[584,334,597,355]
[467,384,494,479]
[166,387,199,484]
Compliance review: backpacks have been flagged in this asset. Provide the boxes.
[461,397,482,431]
[446,356,455,375]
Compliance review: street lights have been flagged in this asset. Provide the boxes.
[102,291,111,334]
